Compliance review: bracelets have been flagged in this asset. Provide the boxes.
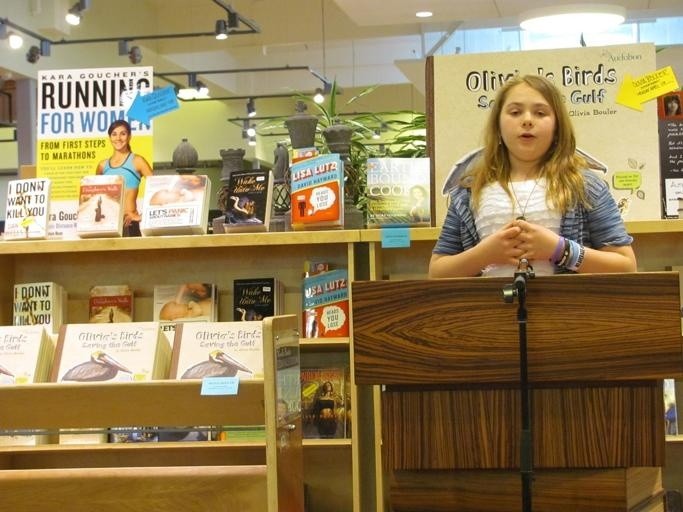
[550,236,586,273]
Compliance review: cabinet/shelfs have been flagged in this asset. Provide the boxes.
[0,313,305,512]
[0,229,360,512]
[360,219,683,512]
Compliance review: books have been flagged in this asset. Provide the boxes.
[367,158,432,228]
[142,176,209,234]
[0,268,351,443]
[224,170,274,232]
[291,155,345,230]
[5,180,48,240]
[78,176,126,237]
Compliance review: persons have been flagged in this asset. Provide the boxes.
[427,74,637,279]
[664,95,682,117]
[96,120,155,237]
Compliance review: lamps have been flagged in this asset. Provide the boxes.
[66,0,87,27]
[119,37,142,64]
[519,3,627,34]
[175,71,210,100]
[243,130,257,146]
[312,83,327,105]
[28,31,51,63]
[246,96,256,118]
[0,20,24,51]
[242,118,258,136]
[215,10,239,42]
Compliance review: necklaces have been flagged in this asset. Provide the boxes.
[507,175,541,222]
